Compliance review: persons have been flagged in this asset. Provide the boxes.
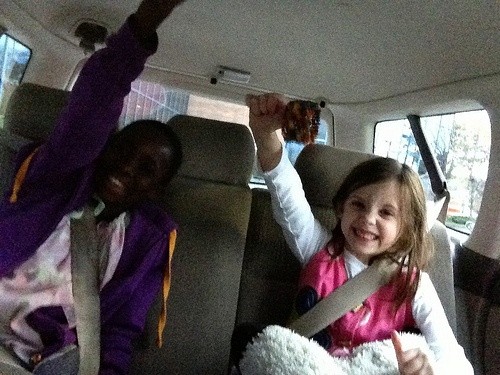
[239,94,474,375]
[1,1,181,375]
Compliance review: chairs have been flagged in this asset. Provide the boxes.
[132,113,255,374]
[230,140,387,374]
[0,80,72,198]
